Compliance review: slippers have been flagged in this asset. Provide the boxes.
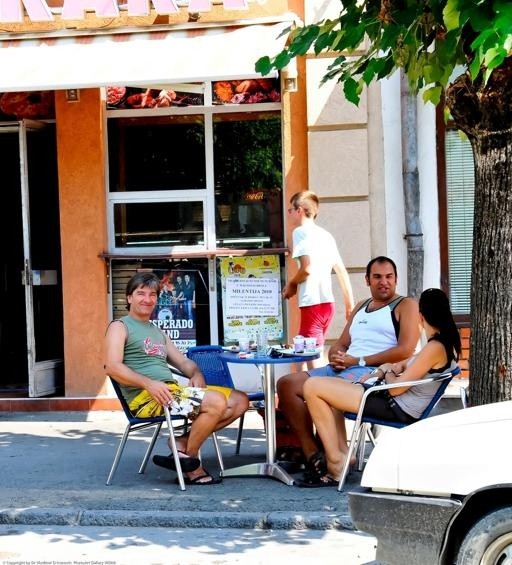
[174,468,222,486]
[276,444,349,488]
[152,449,201,474]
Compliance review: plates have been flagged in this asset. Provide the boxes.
[277,343,318,356]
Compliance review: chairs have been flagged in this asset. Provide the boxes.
[101,345,263,491]
[336,365,460,494]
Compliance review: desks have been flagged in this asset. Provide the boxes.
[219,348,320,488]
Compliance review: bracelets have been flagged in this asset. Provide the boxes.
[378,368,385,375]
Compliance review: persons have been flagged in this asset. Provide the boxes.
[105,273,249,485]
[282,190,354,373]
[160,274,196,319]
[292,288,462,486]
[277,254,422,473]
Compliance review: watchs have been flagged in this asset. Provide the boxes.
[358,357,366,367]
[388,369,398,377]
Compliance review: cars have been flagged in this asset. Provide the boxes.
[347,398,512,560]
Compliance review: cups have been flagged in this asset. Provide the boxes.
[239,338,250,353]
[257,334,268,358]
[293,338,317,353]
